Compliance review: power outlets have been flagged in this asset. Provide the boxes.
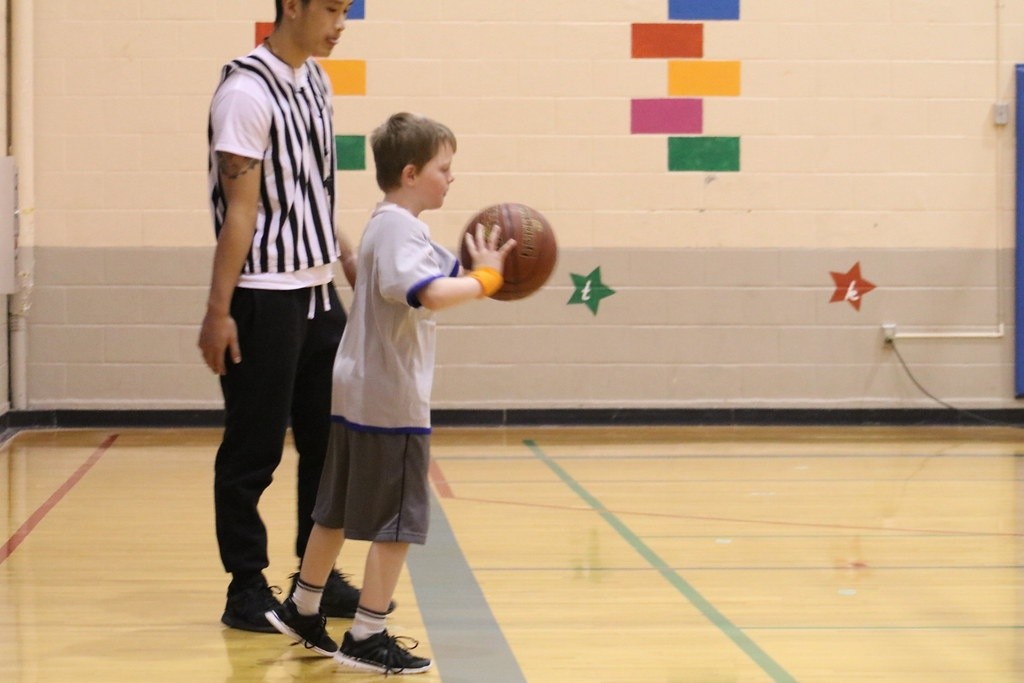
[880,323,896,349]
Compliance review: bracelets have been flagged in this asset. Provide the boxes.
[467,265,504,300]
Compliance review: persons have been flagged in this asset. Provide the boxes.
[263,108,517,677]
[194,0,400,632]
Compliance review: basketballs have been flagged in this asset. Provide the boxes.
[456,198,561,308]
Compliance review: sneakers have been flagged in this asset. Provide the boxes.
[288,568,395,618]
[334,628,432,678]
[221,582,283,632]
[263,596,339,657]
